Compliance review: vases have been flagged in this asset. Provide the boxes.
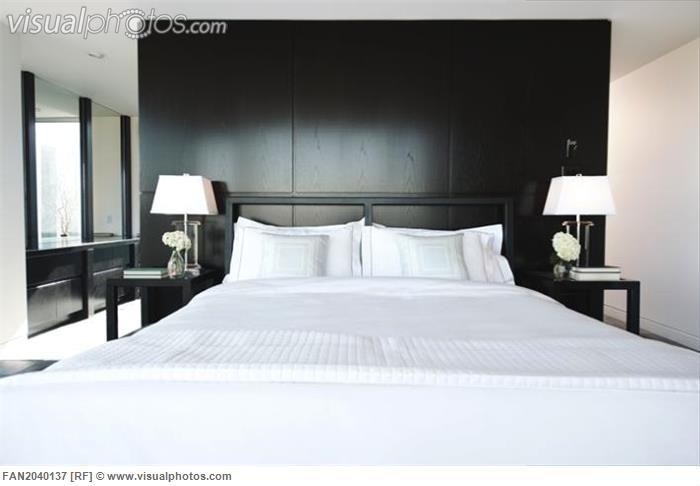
[167,250,186,279]
[551,259,571,283]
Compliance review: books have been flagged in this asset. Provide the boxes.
[121,265,169,281]
[567,265,622,283]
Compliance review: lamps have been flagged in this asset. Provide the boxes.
[543,174,618,267]
[150,172,219,276]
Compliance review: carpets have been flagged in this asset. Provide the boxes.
[1,359,59,378]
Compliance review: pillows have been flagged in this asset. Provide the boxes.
[223,215,515,289]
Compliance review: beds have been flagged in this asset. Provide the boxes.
[0,196,698,463]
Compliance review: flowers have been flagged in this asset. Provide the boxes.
[550,231,582,266]
[161,229,192,277]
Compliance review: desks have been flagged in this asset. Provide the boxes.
[106,269,224,342]
[523,270,640,338]
